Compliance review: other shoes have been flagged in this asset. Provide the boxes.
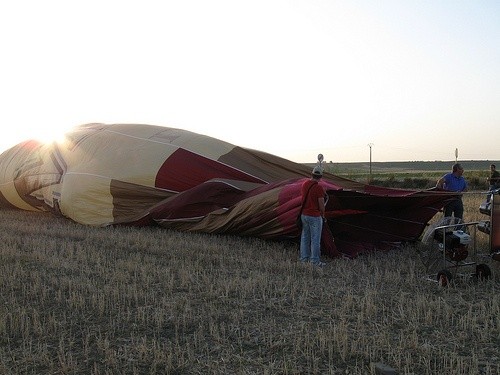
[312,261,326,267]
[301,258,310,263]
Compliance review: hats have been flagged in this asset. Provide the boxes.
[312,166,324,175]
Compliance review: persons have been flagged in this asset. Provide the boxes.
[487,164,500,203]
[436,164,467,231]
[300,165,330,266]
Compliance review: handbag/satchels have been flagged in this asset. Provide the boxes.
[297,215,302,233]
